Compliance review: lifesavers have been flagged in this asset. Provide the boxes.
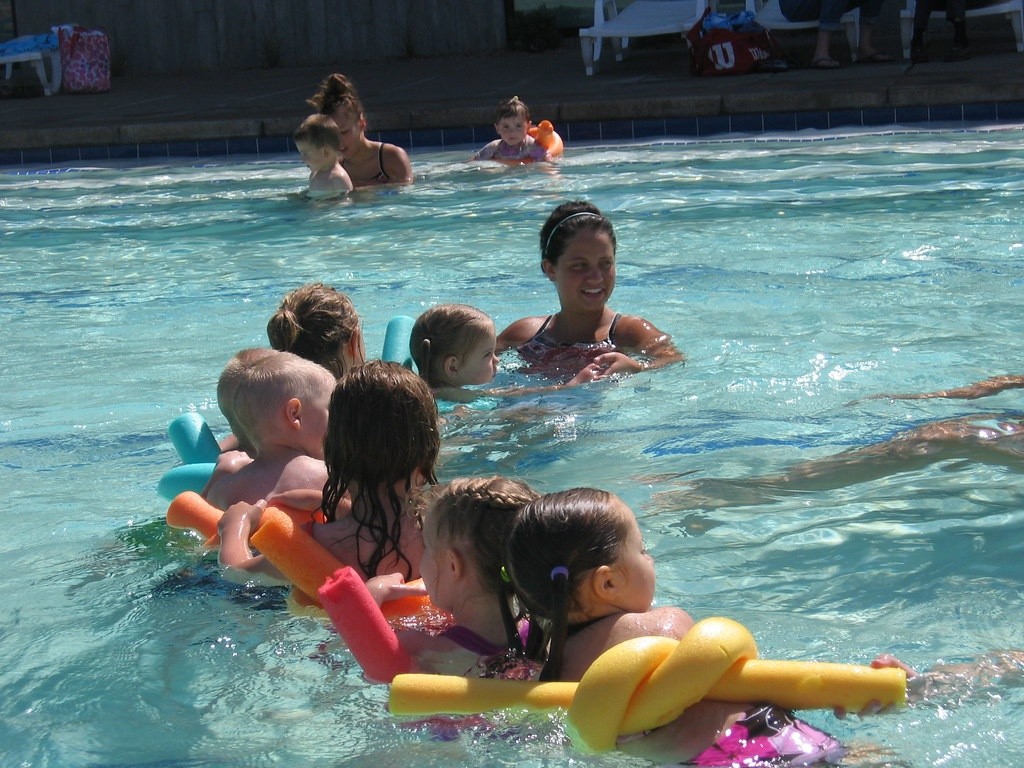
[155,313,420,681]
[383,609,915,757]
[493,119,564,166]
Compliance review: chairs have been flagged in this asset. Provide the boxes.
[578,0,717,77]
[0,33,61,97]
[745,0,860,50]
[900,0,1024,59]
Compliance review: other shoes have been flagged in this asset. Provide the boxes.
[910,46,929,62]
[944,47,968,61]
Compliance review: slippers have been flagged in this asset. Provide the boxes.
[811,58,842,69]
[859,52,894,63]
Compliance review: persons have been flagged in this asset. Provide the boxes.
[496,201,684,376]
[199,284,367,512]
[464,96,555,173]
[506,487,851,762]
[764,0,973,69]
[293,115,353,198]
[305,74,413,187]
[833,650,1024,720]
[409,304,605,406]
[365,476,543,742]
[216,361,442,616]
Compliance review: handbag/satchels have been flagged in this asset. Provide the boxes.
[685,7,780,76]
[56,26,112,92]
